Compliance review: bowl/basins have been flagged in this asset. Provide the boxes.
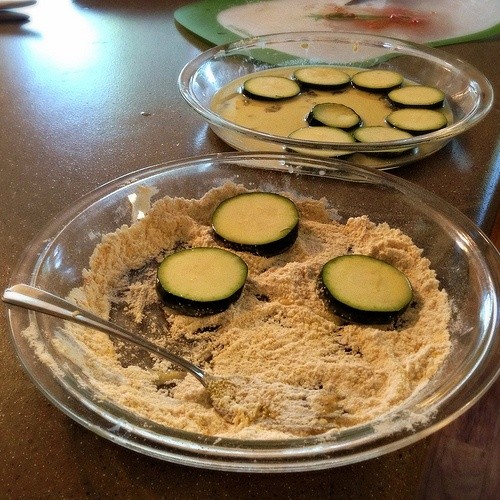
[179,30,494,173]
[3,153,500,474]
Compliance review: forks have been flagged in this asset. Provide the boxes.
[3,284,324,427]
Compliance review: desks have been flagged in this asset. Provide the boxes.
[0,0,500,500]
